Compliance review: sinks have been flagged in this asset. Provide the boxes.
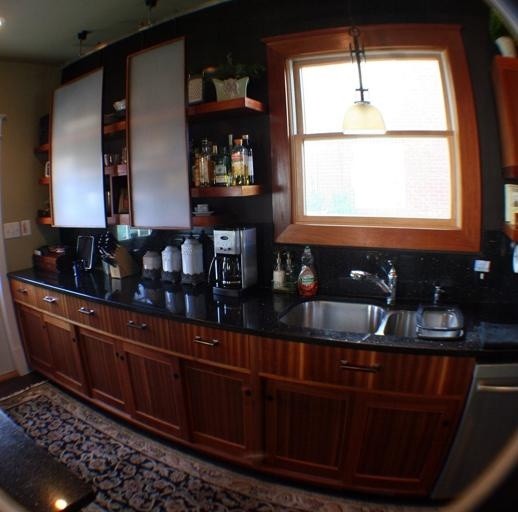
[277,300,386,336]
[382,309,463,340]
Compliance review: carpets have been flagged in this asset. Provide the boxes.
[0,382,438,512]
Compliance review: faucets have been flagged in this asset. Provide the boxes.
[350,259,398,305]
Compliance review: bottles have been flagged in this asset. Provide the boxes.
[213,146,231,186]
[284,251,296,291]
[242,135,254,184]
[190,148,199,187]
[273,251,285,291]
[226,134,233,147]
[296,244,319,299]
[231,139,249,186]
[213,145,218,158]
[199,137,213,187]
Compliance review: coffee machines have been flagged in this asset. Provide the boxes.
[207,223,260,297]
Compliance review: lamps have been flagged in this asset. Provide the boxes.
[342,28,388,134]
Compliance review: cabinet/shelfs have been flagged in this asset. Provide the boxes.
[7,272,518,502]
[33,0,267,230]
